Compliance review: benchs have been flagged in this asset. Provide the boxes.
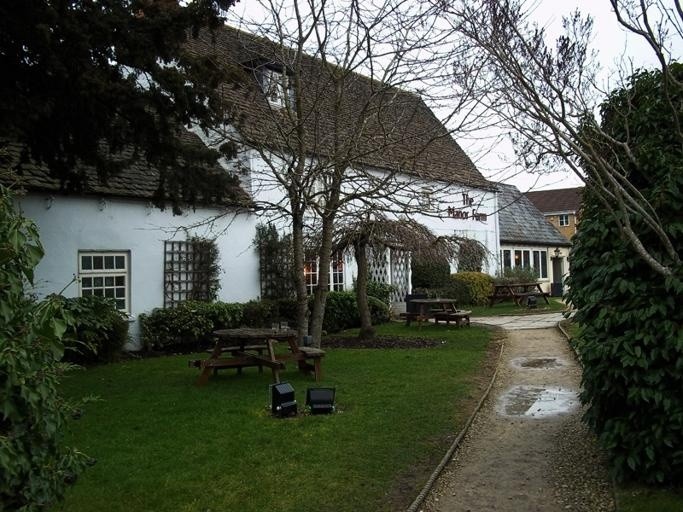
[188,343,326,383]
[400,309,472,329]
[488,291,550,307]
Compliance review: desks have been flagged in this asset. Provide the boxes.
[495,283,542,307]
[214,328,306,385]
[410,298,458,330]
